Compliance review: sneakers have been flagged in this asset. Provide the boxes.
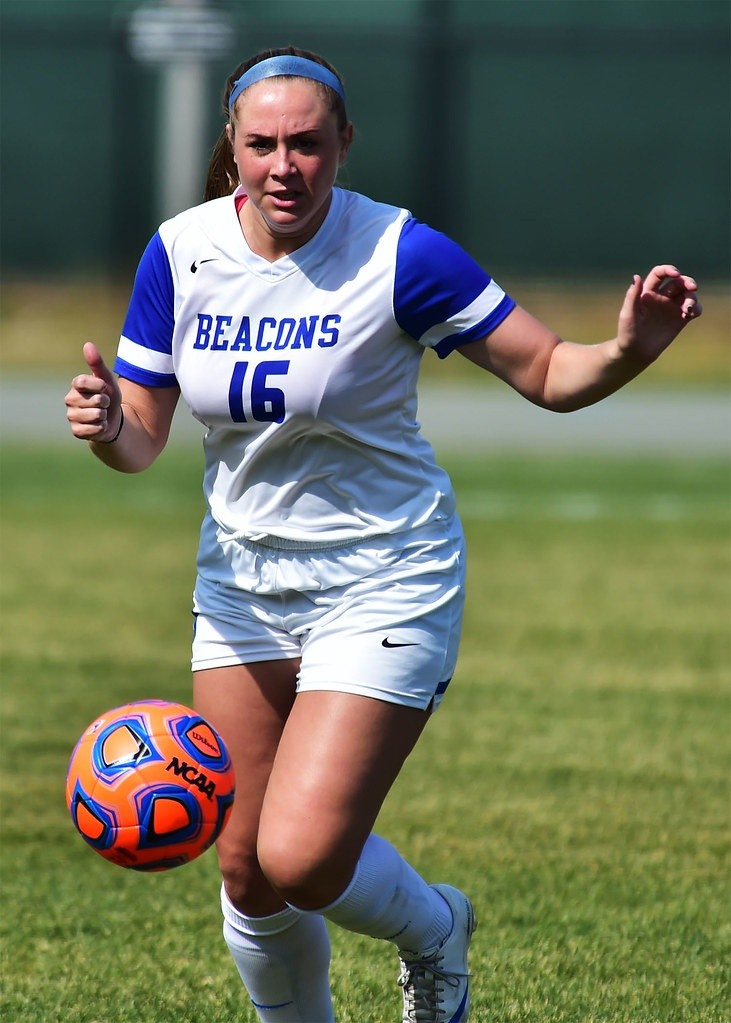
[397,883,477,1022]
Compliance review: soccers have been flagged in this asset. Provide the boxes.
[61,700,237,874]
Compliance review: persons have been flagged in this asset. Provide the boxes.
[61,48,704,1022]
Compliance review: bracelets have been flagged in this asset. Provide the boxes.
[91,402,124,443]
